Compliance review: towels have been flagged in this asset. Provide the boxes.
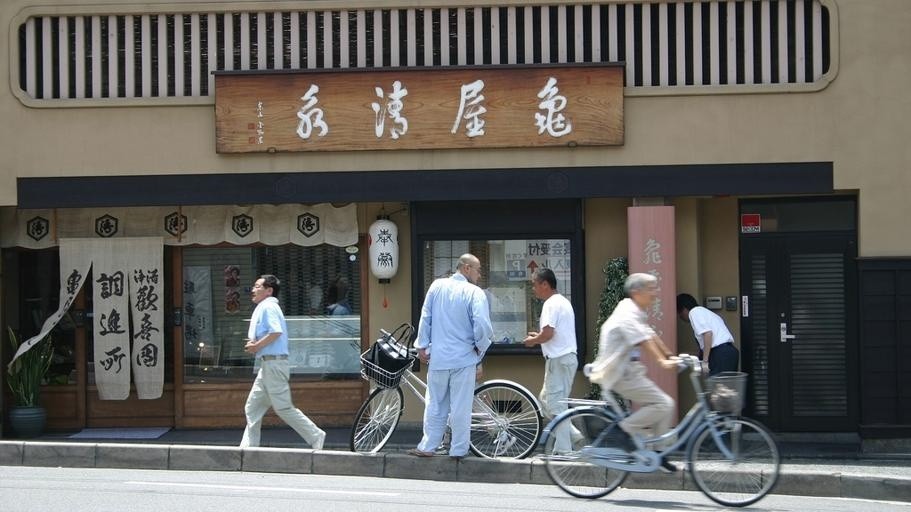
[247,295,280,343]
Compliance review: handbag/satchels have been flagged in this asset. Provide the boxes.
[362,321,416,386]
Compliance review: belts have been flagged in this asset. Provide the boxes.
[259,353,290,362]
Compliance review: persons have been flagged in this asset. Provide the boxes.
[586,272,684,473]
[676,292,740,450]
[410,253,493,457]
[239,275,326,450]
[434,362,482,455]
[523,268,584,462]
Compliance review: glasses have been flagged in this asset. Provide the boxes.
[251,284,262,289]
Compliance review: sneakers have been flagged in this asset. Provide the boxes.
[618,419,647,451]
[311,428,328,451]
[654,451,678,474]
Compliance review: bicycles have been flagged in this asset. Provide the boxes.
[532,354,779,507]
[350,327,545,459]
